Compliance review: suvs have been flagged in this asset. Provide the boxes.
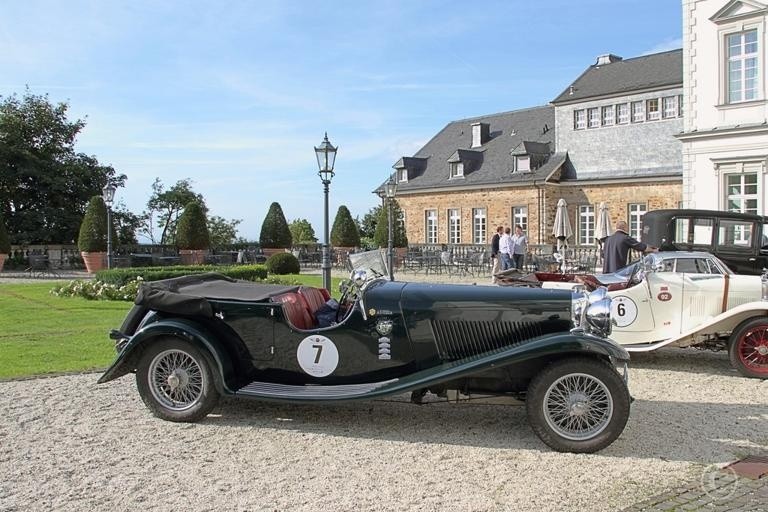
[638,208,767,274]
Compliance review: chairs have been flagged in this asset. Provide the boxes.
[400,243,598,278]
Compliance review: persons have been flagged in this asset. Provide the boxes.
[602,221,659,273]
[499,227,514,270]
[511,224,529,270]
[490,225,504,283]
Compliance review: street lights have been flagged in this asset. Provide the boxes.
[311,131,342,302]
[101,179,116,271]
[385,172,396,282]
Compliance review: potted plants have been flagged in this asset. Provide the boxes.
[78,195,117,275]
[330,205,363,266]
[259,201,298,265]
[0,208,12,273]
[174,201,210,263]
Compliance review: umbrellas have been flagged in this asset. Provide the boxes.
[594,201,614,251]
[552,198,573,274]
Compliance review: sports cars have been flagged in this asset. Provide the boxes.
[497,253,768,385]
[96,272,640,456]
